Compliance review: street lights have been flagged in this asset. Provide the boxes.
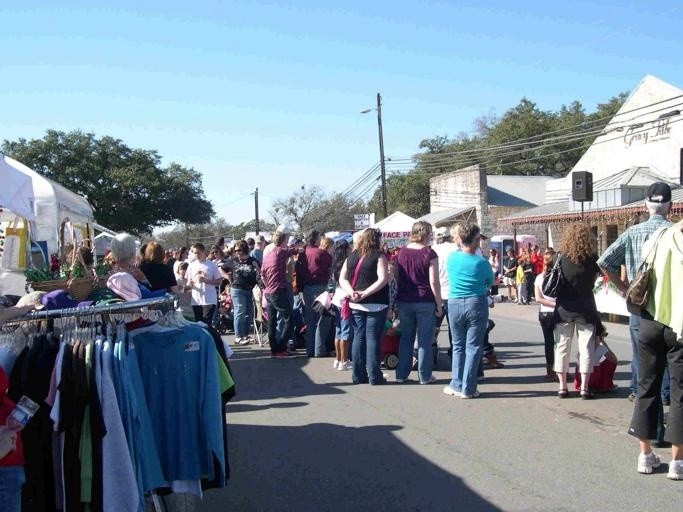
[359,93,388,218]
[249,188,260,235]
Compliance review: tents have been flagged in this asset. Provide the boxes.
[353,212,437,247]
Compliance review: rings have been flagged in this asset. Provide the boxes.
[9,436,14,444]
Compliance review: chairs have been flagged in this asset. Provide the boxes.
[252,297,270,347]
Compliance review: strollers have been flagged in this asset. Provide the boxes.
[216,293,234,335]
[378,317,399,370]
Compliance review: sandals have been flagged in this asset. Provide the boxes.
[245,336,256,344]
[234,337,250,346]
[558,389,568,399]
[580,390,594,400]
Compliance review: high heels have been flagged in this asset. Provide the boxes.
[487,355,504,369]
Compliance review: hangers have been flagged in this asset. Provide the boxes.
[0,298,196,359]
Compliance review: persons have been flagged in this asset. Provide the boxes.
[339,228,389,385]
[444,223,494,398]
[383,221,504,384]
[63,232,177,302]
[0,363,29,512]
[624,216,682,481]
[503,242,553,306]
[536,250,570,379]
[0,303,36,325]
[594,179,674,408]
[260,224,352,371]
[164,235,267,344]
[553,221,602,399]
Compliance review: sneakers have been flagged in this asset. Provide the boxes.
[443,386,460,396]
[333,359,341,368]
[461,390,480,399]
[337,359,352,371]
[395,375,408,383]
[275,351,296,359]
[544,371,558,381]
[419,375,436,385]
[637,451,660,473]
[667,460,682,480]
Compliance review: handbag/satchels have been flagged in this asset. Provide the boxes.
[340,298,349,320]
[625,229,666,308]
[573,338,616,393]
[541,253,565,298]
[311,290,336,320]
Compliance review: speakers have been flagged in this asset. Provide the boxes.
[572,171,593,201]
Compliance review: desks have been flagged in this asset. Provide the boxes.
[593,277,630,322]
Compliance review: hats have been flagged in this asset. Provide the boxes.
[434,227,449,238]
[276,224,290,233]
[107,271,142,303]
[139,283,167,298]
[89,287,125,307]
[646,183,671,204]
[42,289,95,309]
[287,236,297,246]
[256,234,265,243]
[14,291,46,310]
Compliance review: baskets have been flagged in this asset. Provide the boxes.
[31,217,92,301]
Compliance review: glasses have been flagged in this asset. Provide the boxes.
[212,250,218,254]
[234,249,241,253]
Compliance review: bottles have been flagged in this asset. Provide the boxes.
[4,394,37,432]
